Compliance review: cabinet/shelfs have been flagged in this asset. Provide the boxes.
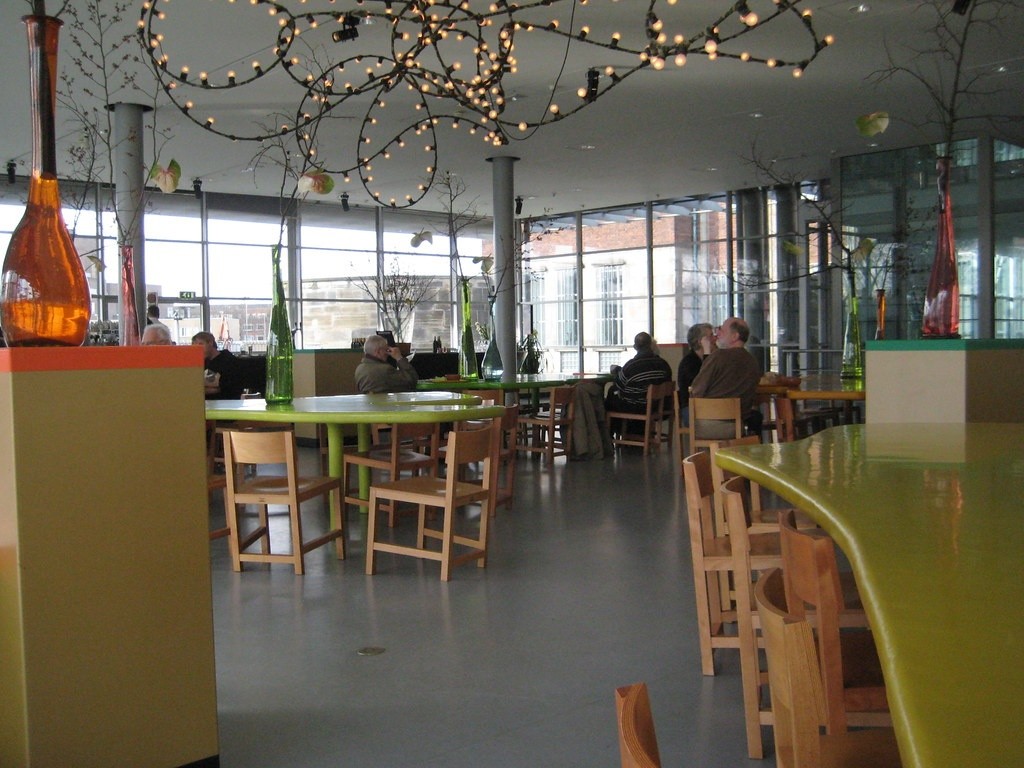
[865,337,1024,426]
[0,344,222,768]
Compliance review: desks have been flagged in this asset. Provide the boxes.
[759,369,866,443]
[714,420,1024,768]
[204,389,507,541]
[417,371,613,460]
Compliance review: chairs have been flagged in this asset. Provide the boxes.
[201,363,902,768]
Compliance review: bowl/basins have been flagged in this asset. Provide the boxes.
[445,375,460,380]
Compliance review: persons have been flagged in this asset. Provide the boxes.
[677,322,764,433]
[605,331,672,445]
[687,315,762,490]
[191,330,237,445]
[355,334,452,463]
[140,304,170,345]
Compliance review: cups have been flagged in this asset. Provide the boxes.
[478,379,485,384]
[249,346,252,354]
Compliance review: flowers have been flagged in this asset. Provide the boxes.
[475,322,490,340]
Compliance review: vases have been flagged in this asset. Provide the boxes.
[457,278,479,382]
[264,244,295,406]
[874,289,888,340]
[481,295,504,383]
[0,14,94,347]
[922,155,964,340]
[516,350,529,374]
[396,343,411,353]
[841,273,866,379]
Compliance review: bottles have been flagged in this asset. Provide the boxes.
[351,338,366,349]
[91,320,118,329]
[433,336,441,353]
[87,331,119,346]
[474,340,488,352]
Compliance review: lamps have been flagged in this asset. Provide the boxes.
[193,177,205,199]
[341,192,350,211]
[515,196,523,214]
[332,14,360,43]
[7,163,17,185]
[586,71,599,102]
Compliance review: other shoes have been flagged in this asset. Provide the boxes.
[214,463,223,474]
[424,453,445,464]
